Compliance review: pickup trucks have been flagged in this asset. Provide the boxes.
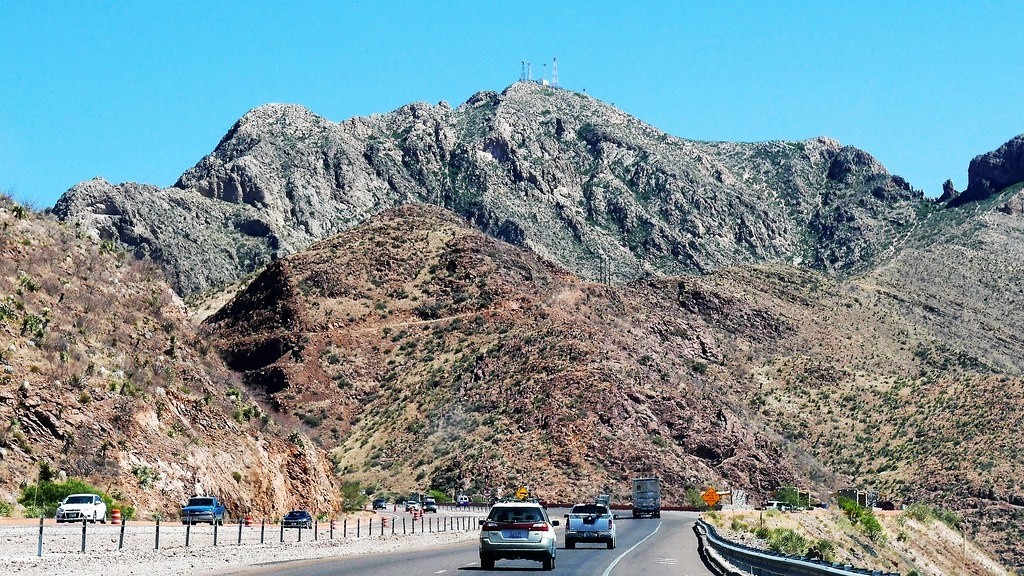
[564,500,619,549]
[181,496,226,526]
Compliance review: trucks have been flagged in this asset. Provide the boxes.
[422,494,437,513]
[632,478,661,518]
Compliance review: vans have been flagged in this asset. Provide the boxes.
[456,494,470,507]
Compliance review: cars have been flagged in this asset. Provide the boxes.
[56,494,108,524]
[406,501,420,512]
[372,500,388,509]
[281,510,313,529]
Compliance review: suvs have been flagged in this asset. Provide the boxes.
[479,499,559,571]
